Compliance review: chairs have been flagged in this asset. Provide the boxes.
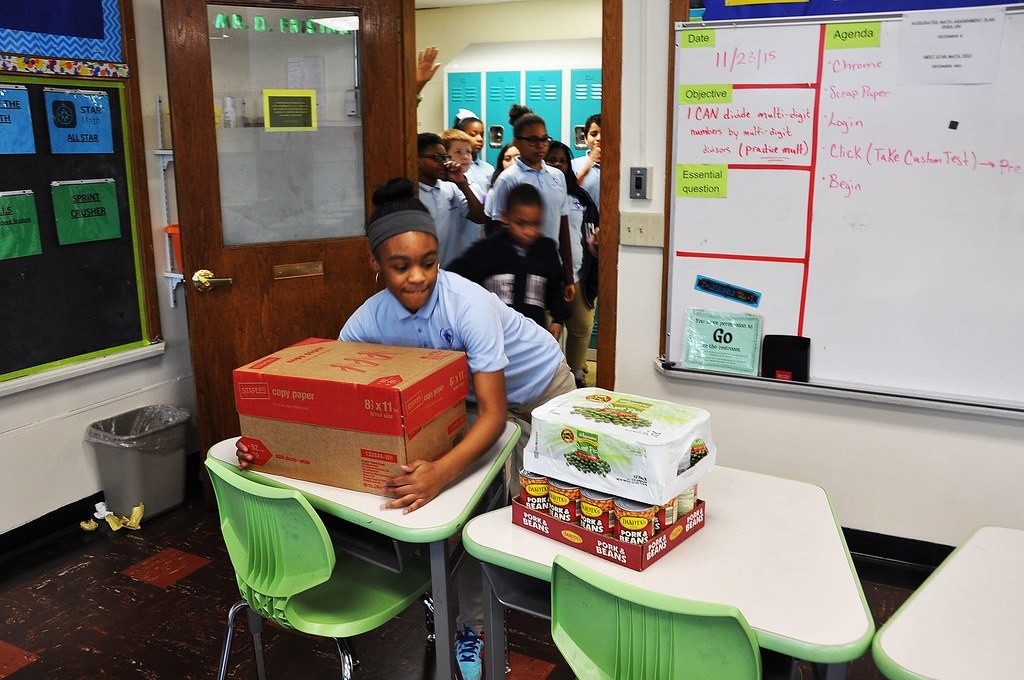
[200,457,432,680]
[546,553,765,680]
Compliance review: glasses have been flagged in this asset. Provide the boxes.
[422,153,452,163]
[516,134,553,147]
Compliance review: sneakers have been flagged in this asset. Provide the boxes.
[453,630,483,680]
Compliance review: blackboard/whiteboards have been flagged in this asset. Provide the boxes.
[665,3,1024,423]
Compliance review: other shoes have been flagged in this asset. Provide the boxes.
[573,373,590,388]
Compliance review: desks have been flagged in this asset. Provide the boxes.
[461,462,876,680]
[868,522,1024,680]
[202,414,522,679]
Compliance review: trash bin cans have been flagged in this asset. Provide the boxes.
[86,404,191,523]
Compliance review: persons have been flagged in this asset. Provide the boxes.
[416,45,601,389]
[236,176,578,680]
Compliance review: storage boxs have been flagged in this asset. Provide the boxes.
[511,493,706,573]
[232,334,473,499]
